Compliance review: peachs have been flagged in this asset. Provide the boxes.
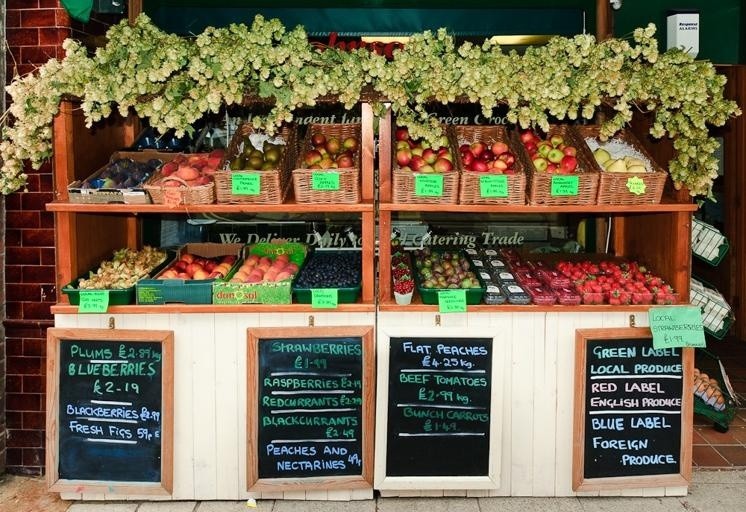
[231,253,297,283]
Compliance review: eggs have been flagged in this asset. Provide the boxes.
[694,367,724,405]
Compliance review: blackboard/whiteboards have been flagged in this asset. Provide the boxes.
[246,325,374,491]
[46,327,174,496]
[386,336,493,476]
[572,327,695,491]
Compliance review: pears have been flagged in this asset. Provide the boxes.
[592,147,647,174]
[229,136,284,170]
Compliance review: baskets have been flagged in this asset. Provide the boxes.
[142,120,361,204]
[391,124,668,206]
[693,374,737,428]
[690,273,736,340]
[691,218,729,267]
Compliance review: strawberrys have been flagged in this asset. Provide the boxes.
[558,259,675,304]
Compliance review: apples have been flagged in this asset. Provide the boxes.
[395,125,577,175]
[156,253,234,279]
[162,148,223,186]
[305,133,357,169]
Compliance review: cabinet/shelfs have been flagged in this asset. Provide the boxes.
[54,90,373,310]
[385,92,688,309]
